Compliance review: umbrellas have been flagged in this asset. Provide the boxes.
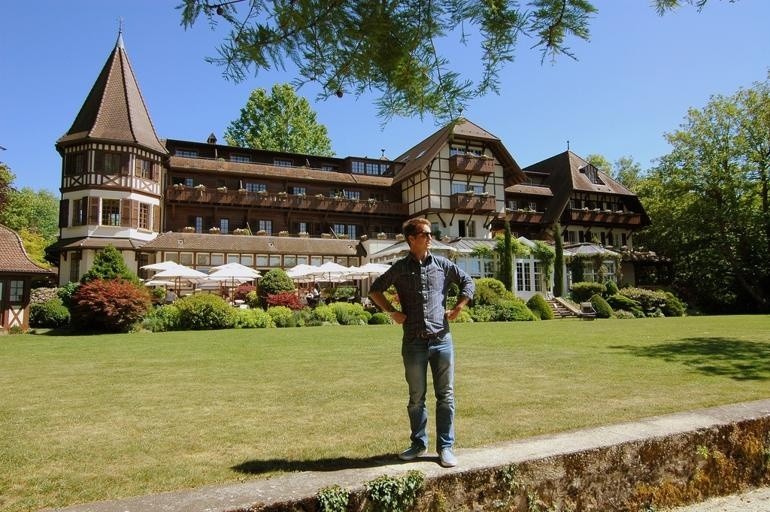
[139,258,392,303]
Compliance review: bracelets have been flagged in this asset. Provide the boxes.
[387,306,394,315]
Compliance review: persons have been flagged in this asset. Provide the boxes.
[367,216,475,467]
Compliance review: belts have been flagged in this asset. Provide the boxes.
[415,331,439,338]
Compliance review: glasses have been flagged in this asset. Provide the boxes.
[414,230,433,237]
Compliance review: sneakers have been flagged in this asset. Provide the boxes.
[399,447,428,460]
[437,448,457,467]
